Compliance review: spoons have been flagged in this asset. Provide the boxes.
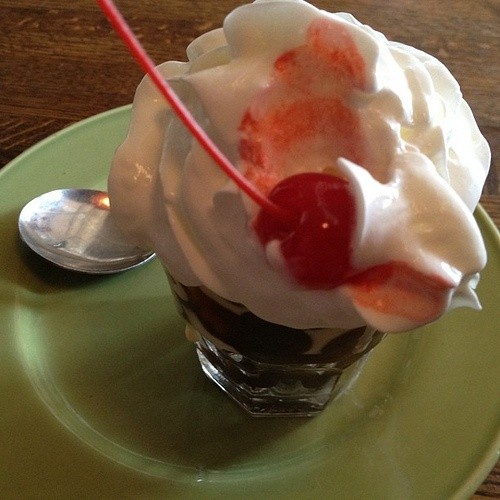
[18,188,156,276]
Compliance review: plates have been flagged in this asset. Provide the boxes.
[1,105,500,500]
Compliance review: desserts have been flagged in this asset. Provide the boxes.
[97,0,492,334]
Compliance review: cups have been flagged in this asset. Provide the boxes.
[162,262,390,420]
